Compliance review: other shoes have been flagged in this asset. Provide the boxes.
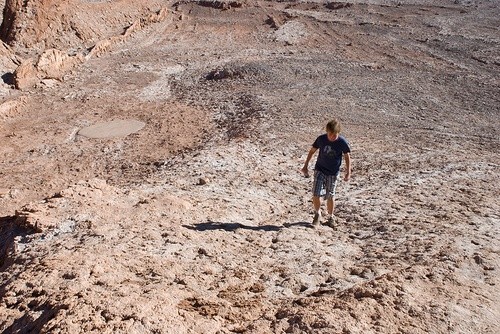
[312,213,320,225]
[328,218,337,227]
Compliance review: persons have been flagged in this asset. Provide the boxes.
[301,120,351,227]
